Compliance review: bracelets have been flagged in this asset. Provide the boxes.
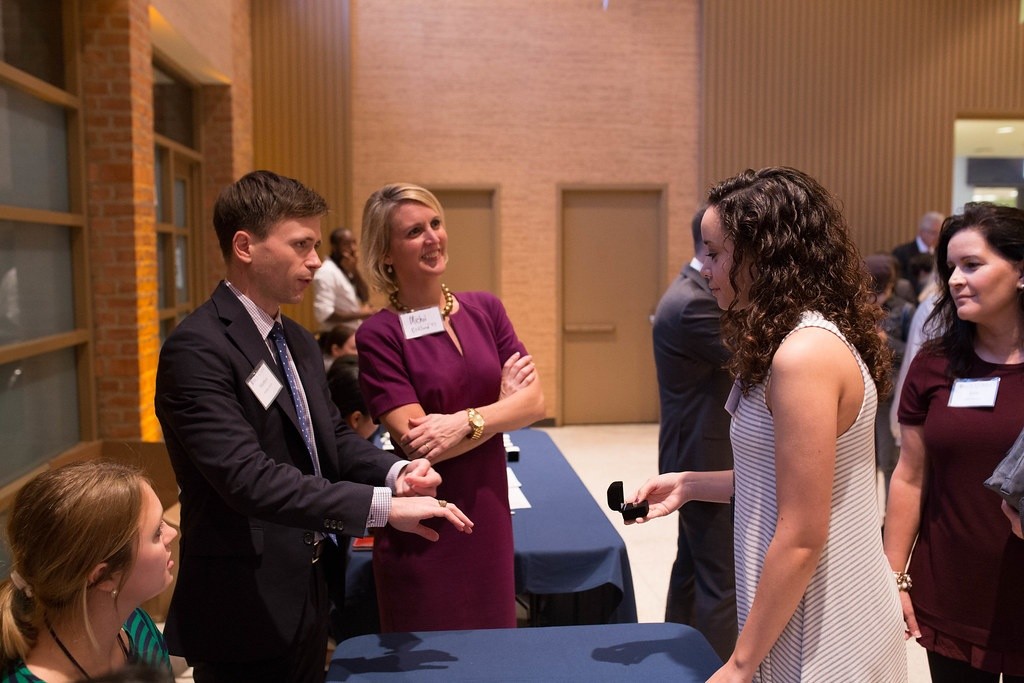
[893,572,912,592]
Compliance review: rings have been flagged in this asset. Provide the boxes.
[439,500,447,507]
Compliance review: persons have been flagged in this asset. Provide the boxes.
[652,208,739,667]
[0,457,178,683]
[863,212,953,546]
[623,166,908,683]
[326,354,380,440]
[883,202,1024,683]
[355,183,547,633]
[153,171,474,683]
[317,325,358,372]
[313,228,382,333]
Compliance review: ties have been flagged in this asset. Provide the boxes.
[266,321,333,539]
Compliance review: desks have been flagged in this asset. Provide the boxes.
[345,429,640,632]
[324,620,724,683]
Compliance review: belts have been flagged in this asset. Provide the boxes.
[309,536,333,566]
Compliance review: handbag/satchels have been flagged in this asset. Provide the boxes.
[983,428,1024,512]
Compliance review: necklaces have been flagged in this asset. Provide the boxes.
[45,620,130,679]
[389,282,452,319]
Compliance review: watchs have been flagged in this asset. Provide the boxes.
[466,408,485,441]
